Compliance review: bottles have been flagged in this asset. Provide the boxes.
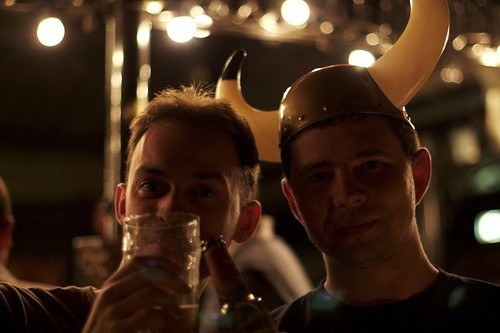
[202,235,282,333]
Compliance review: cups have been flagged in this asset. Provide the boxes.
[121,214,201,323]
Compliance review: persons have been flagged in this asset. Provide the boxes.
[215,64,500,333]
[0,79,281,333]
